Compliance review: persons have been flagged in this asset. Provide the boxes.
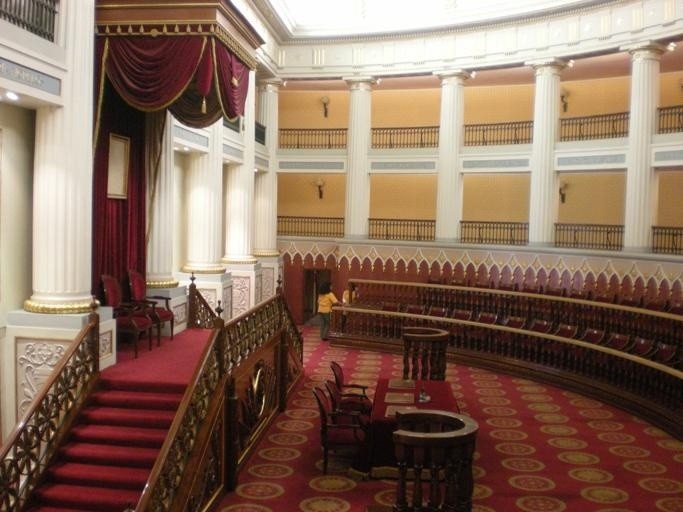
[315,283,338,341]
[341,282,360,330]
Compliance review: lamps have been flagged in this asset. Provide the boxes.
[559,181,567,201]
[320,96,332,117]
[558,88,572,109]
[316,178,328,199]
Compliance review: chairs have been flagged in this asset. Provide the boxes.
[379,275,683,400]
[101,274,153,360]
[312,360,374,477]
[126,267,175,348]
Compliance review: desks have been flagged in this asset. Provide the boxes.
[370,374,464,473]
